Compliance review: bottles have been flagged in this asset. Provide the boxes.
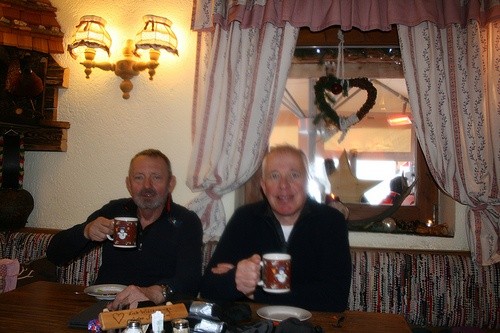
[171,318,190,333]
[122,320,143,333]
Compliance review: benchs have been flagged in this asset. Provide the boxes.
[0,228,500,328]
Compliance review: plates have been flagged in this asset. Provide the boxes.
[84,284,128,300]
[256,306,312,322]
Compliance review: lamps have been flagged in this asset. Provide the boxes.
[67,13,180,100]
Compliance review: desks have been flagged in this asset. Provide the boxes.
[0,280,412,333]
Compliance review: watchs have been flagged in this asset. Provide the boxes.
[160,284,173,304]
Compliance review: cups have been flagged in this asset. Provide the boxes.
[106,217,138,248]
[257,253,291,294]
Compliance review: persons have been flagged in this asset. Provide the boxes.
[378,177,415,206]
[199,145,352,313]
[46,149,204,315]
